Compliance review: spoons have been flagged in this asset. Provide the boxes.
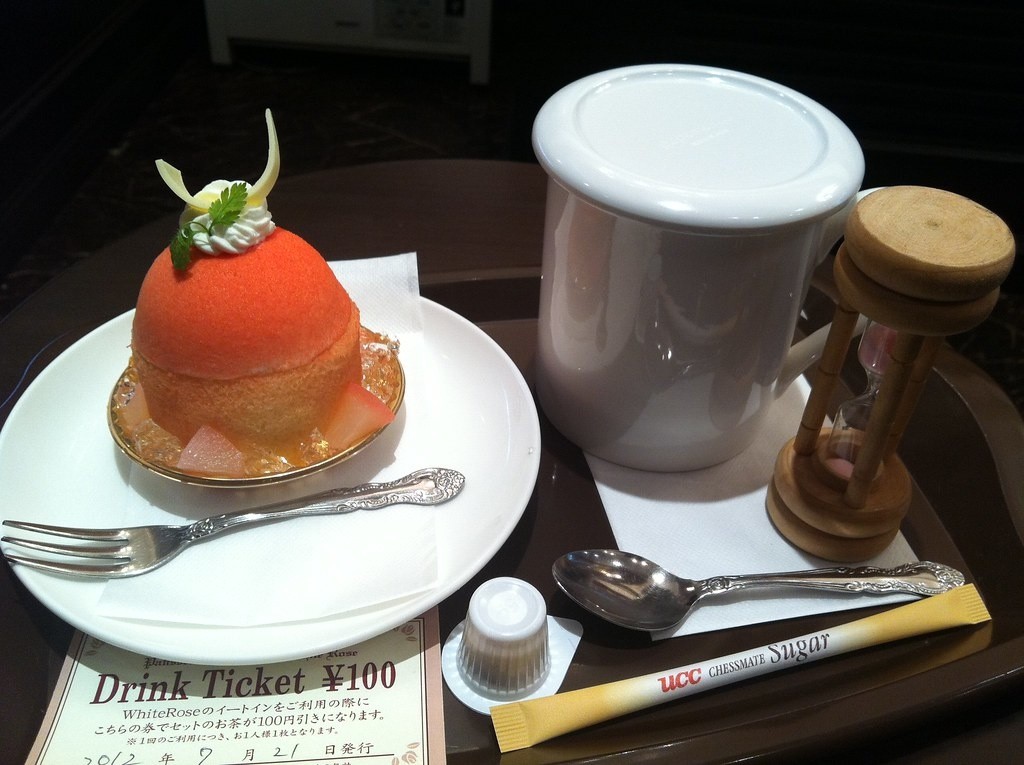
[553,545,966,633]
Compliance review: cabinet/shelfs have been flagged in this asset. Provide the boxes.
[206,1,492,81]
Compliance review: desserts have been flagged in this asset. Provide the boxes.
[114,107,401,477]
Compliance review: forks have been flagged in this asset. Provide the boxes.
[0,466,467,581]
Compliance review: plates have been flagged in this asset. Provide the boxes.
[111,324,406,487]
[0,272,543,668]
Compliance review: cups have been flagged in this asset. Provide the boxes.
[532,59,886,473]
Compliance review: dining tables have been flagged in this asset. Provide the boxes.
[1,155,1024,765]
